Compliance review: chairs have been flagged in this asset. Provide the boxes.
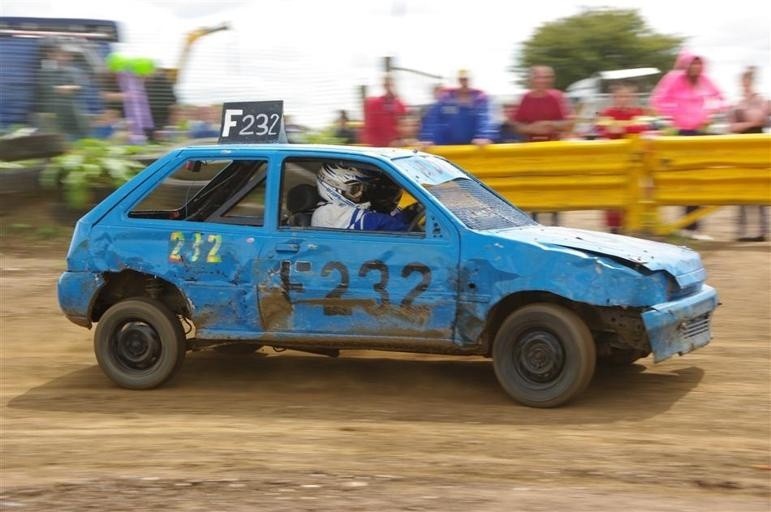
[287,180,323,232]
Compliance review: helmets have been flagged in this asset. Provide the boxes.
[316,160,384,209]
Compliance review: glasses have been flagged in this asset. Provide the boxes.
[317,167,364,199]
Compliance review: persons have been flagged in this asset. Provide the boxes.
[725,65,771,245]
[648,40,732,239]
[310,157,427,232]
[361,167,405,215]
[497,99,532,146]
[514,66,577,229]
[35,41,178,142]
[596,83,657,232]
[420,69,501,144]
[363,70,408,146]
[387,109,434,145]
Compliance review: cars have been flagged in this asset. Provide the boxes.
[52,142,717,412]
